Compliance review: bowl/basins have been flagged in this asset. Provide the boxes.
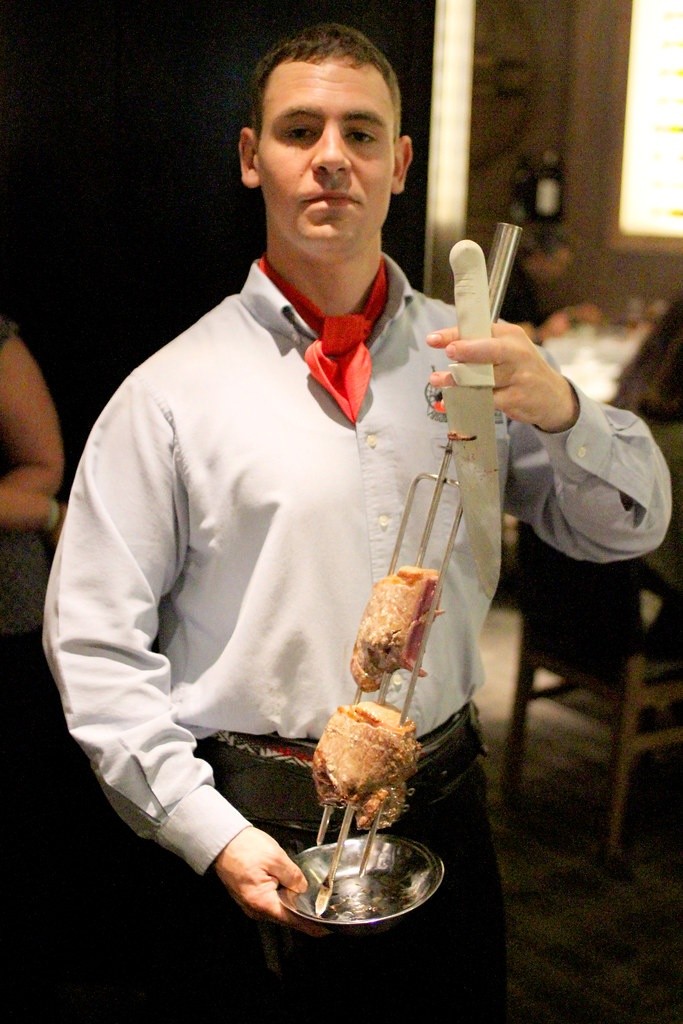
[276,833,444,934]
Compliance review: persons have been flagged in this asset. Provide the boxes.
[43,24,675,1024]
[0,304,67,714]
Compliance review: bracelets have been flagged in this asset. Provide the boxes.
[48,497,58,531]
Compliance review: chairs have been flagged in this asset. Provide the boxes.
[502,519,683,870]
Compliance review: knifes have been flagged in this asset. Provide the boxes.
[448,237,503,599]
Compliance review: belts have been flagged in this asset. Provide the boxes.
[196,702,487,834]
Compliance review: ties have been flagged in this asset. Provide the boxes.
[261,253,389,426]
